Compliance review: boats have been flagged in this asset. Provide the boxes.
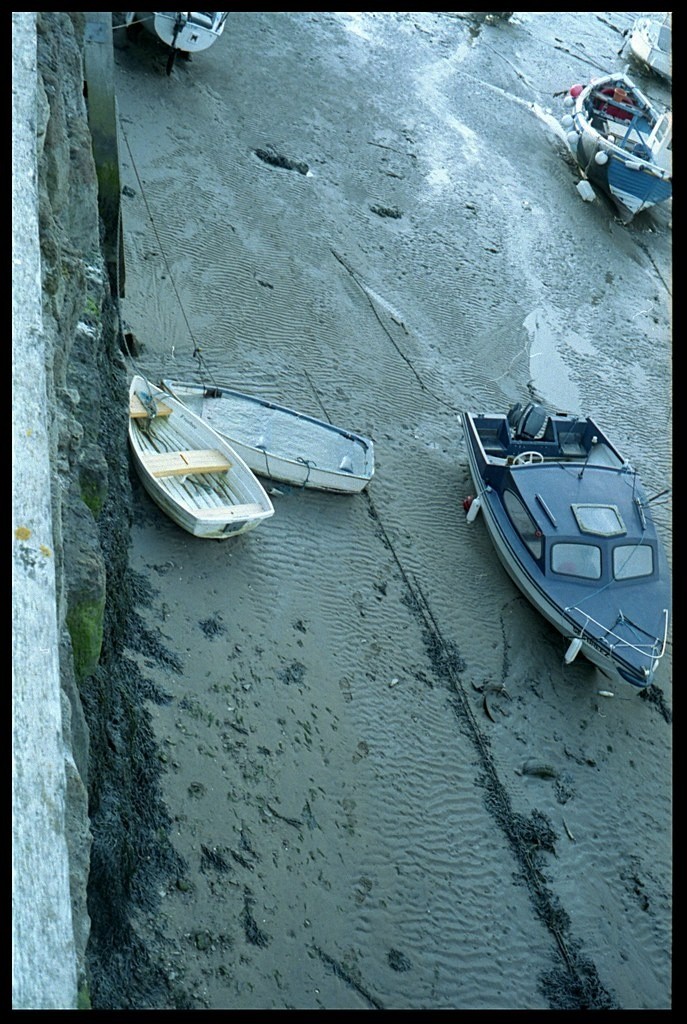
[111,12,231,66]
[628,12,672,88]
[561,64,673,225]
[126,370,276,544]
[160,379,376,499]
[455,404,672,692]
